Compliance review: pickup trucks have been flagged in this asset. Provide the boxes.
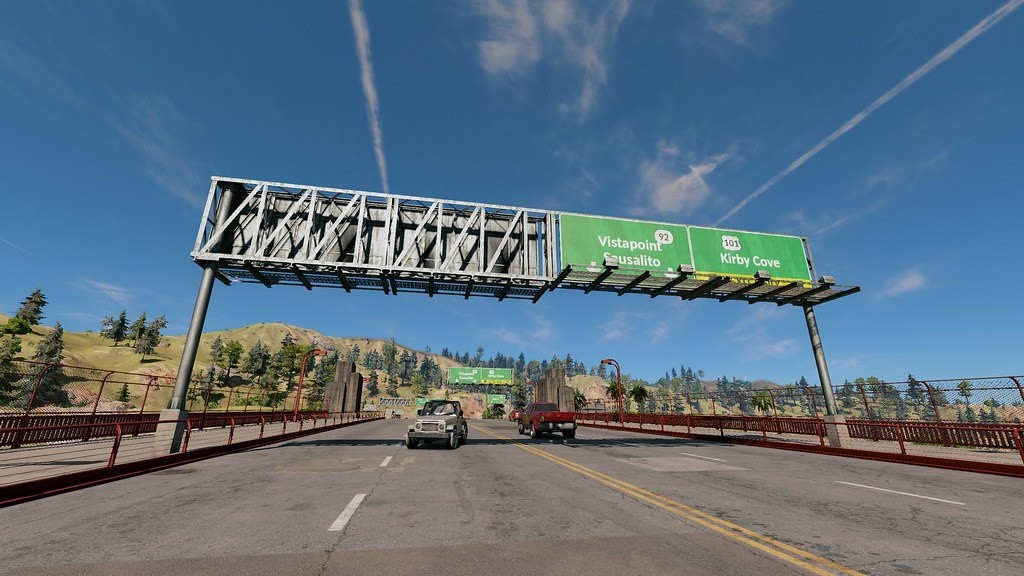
[517,402,578,439]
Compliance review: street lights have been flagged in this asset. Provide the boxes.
[601,359,623,424]
[291,349,327,422]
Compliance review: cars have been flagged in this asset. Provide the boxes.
[509,406,521,422]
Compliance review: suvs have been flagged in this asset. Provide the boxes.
[406,400,468,449]
